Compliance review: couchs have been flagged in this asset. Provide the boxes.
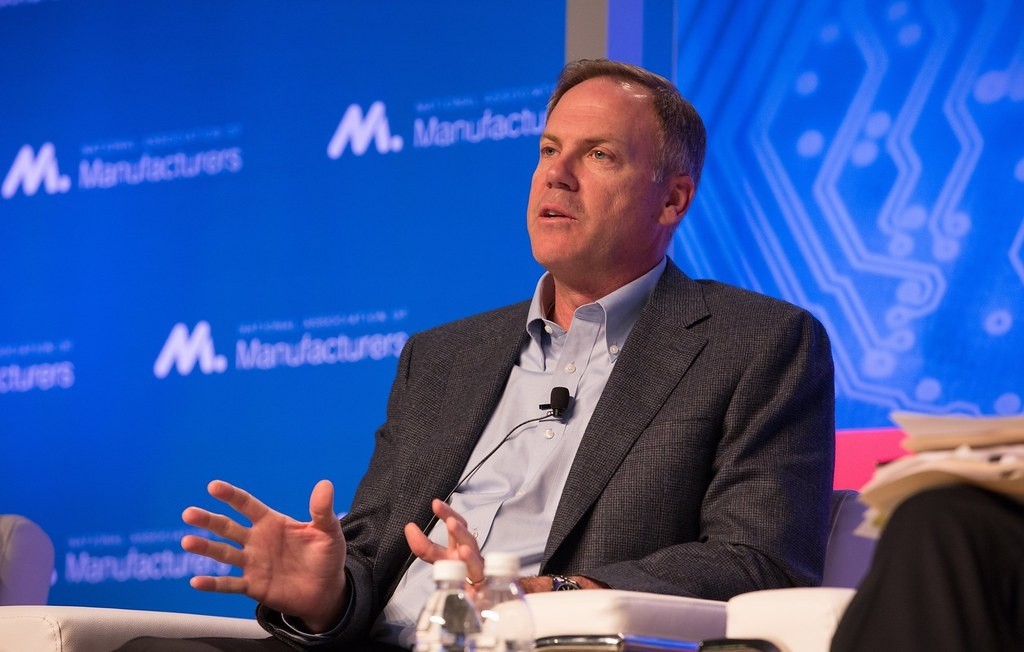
[1,489,879,652]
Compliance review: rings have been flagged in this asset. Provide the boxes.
[466,577,487,586]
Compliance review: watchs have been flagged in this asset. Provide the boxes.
[549,574,582,591]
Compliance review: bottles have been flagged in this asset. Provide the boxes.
[412,561,486,652]
[467,553,534,652]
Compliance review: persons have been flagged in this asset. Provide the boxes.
[120,56,836,652]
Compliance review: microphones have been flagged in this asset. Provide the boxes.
[551,386,570,417]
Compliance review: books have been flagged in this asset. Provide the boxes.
[857,409,1024,537]
